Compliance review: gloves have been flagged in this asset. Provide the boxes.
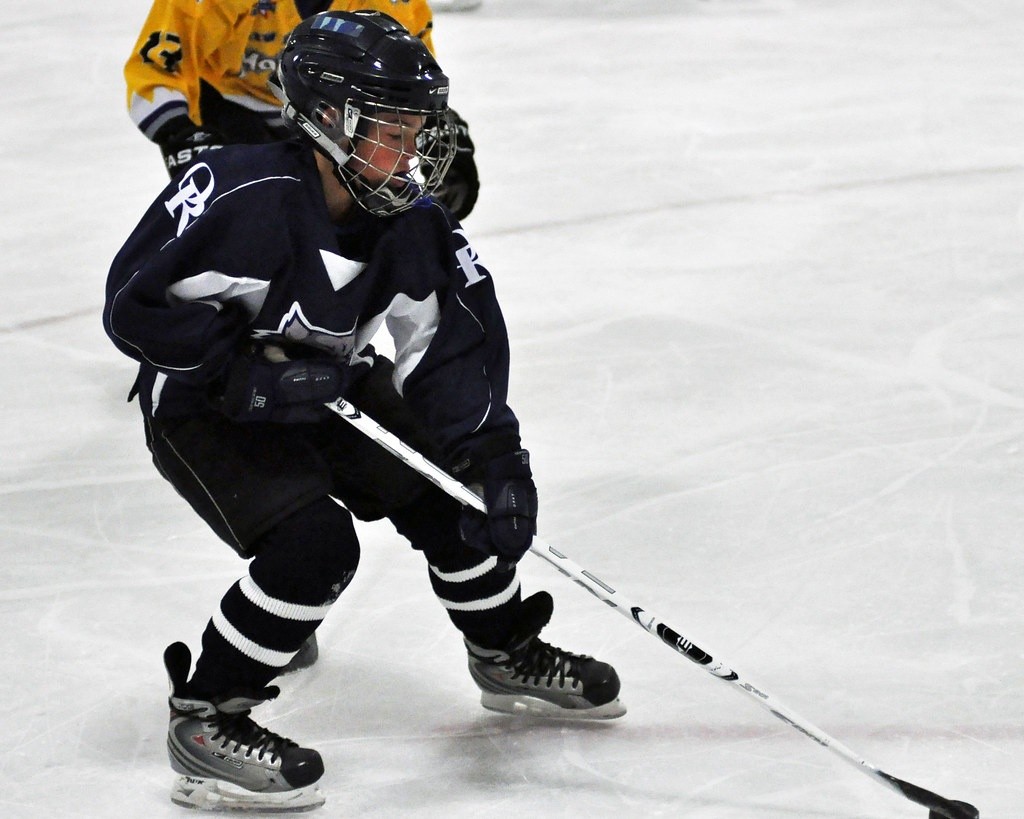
[414,106,479,221]
[203,334,354,425]
[440,422,537,561]
[138,101,222,180]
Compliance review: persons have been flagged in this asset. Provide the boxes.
[102,0,627,814]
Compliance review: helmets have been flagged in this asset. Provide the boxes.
[266,9,458,218]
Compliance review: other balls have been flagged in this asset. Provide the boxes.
[929,799,979,819]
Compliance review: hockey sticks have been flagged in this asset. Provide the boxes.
[322,393,979,819]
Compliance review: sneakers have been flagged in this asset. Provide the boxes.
[463,591,627,721]
[163,641,327,812]
